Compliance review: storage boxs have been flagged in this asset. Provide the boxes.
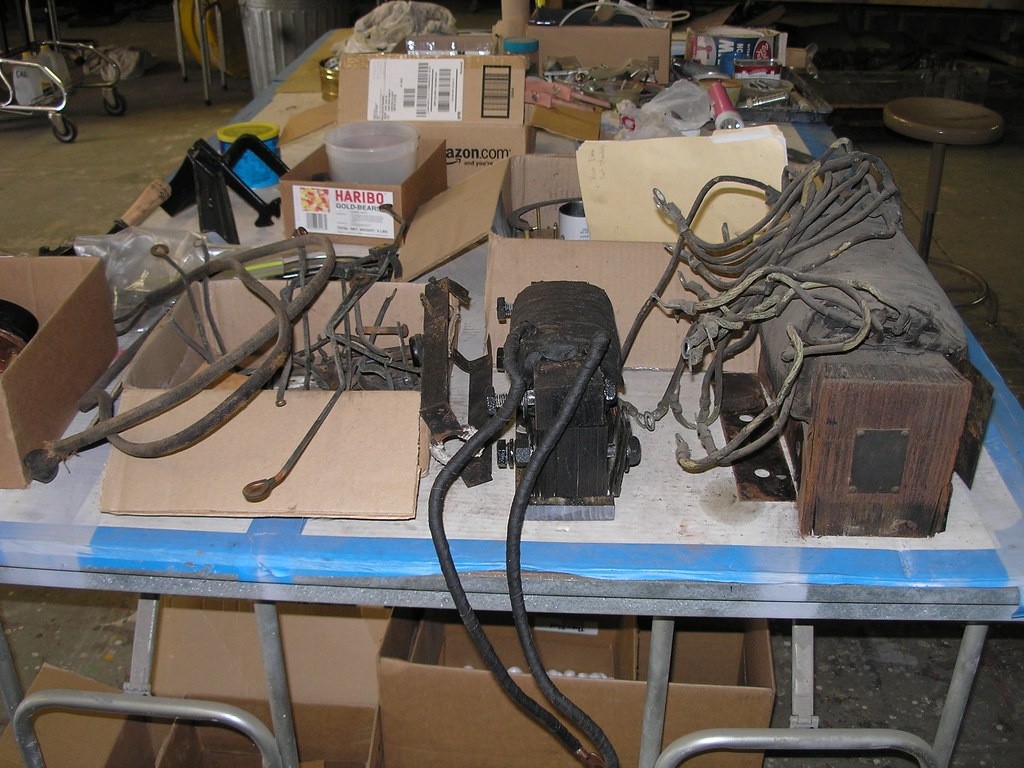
[0,7,789,522]
[0,583,777,768]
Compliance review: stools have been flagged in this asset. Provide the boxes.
[879,97,1006,329]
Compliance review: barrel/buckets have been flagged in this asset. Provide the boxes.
[323,120,421,186]
[740,78,793,107]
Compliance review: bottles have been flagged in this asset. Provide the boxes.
[13,51,43,105]
[37,45,72,93]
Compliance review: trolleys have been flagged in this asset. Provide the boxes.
[0,0,127,143]
